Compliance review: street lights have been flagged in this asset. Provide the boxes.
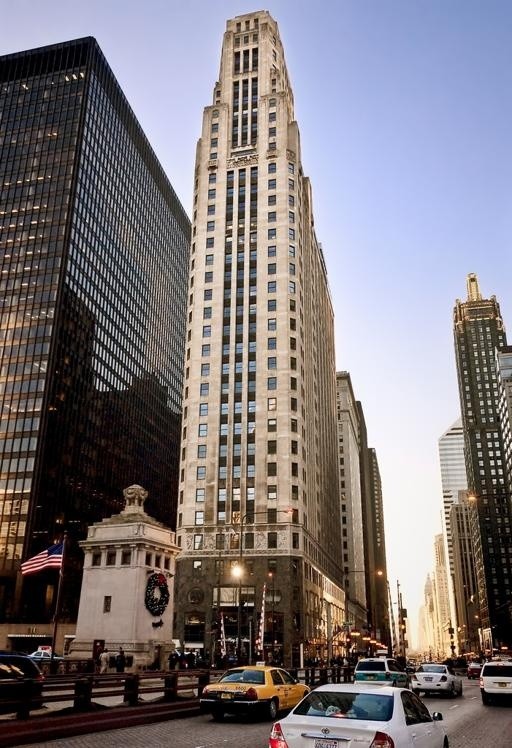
[238,510,298,665]
[345,570,387,626]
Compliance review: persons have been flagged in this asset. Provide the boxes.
[168,647,196,670]
[115,650,125,672]
[213,643,223,669]
[98,647,109,673]
[305,651,369,668]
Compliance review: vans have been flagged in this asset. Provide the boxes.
[354,656,409,689]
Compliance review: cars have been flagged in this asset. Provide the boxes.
[200,665,311,721]
[268,683,449,748]
[27,650,64,663]
[411,664,462,699]
[467,661,512,705]
[0,655,46,719]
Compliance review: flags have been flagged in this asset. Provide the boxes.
[20,540,65,576]
